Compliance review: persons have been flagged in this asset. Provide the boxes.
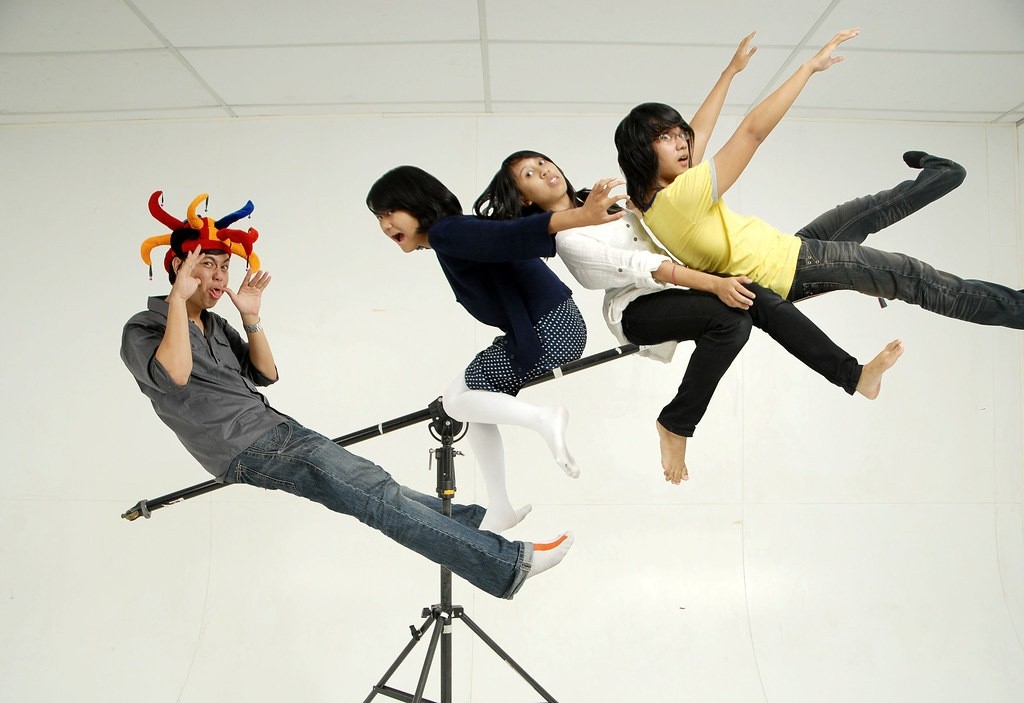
[615,29,1023,330]
[120,216,573,599]
[365,166,586,538]
[473,150,903,485]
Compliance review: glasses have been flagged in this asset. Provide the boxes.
[652,130,690,144]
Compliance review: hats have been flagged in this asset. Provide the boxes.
[141,190,261,281]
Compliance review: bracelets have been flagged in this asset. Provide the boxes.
[240,318,263,332]
